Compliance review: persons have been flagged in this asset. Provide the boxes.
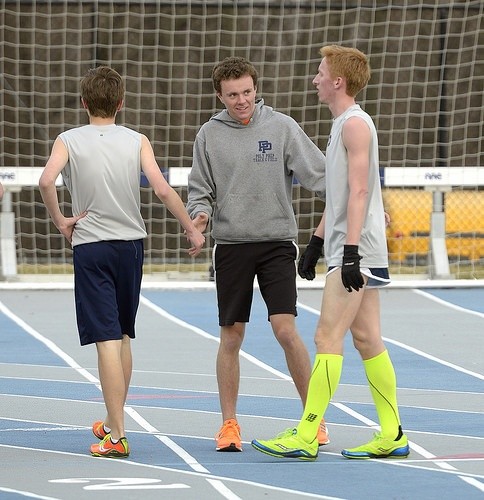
[39,66,205,458]
[188,57,329,452]
[250,45,410,460]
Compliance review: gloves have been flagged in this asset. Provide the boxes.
[342,244,365,293]
[298,235,324,280]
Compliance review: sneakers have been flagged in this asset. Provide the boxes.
[215,419,244,453]
[91,434,130,459]
[318,418,331,445]
[92,420,111,439]
[251,427,319,462]
[340,432,410,458]
